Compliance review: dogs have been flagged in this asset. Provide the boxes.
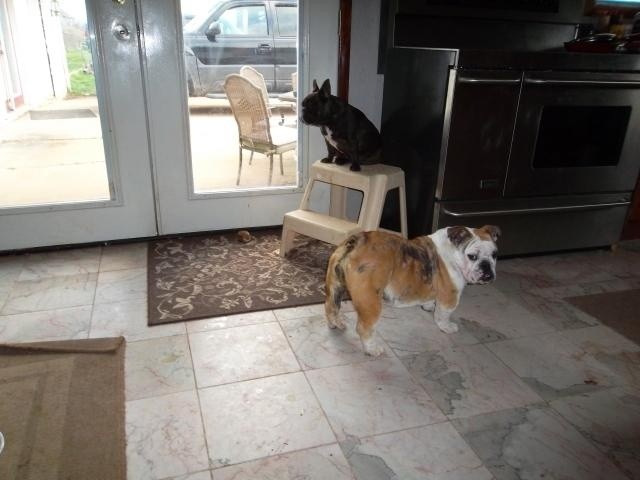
[321,222,504,359]
[297,78,385,173]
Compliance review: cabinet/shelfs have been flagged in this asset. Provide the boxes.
[382,47,640,253]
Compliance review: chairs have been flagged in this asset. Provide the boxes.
[222,65,299,189]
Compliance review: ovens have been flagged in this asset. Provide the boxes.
[501,70,640,199]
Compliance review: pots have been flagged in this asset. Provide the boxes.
[563,29,640,54]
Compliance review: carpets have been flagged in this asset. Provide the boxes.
[0,338,125,479]
[147,229,355,325]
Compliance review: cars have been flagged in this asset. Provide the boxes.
[183,0,298,99]
